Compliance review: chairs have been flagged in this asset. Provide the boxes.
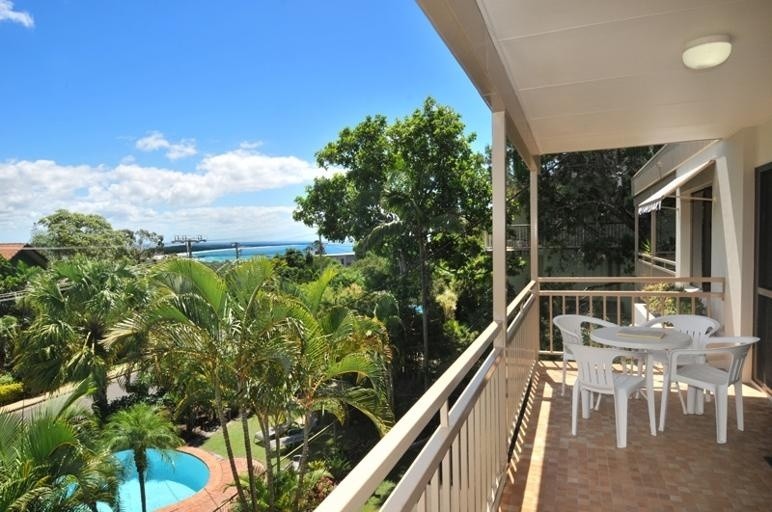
[658,337,759,446]
[255,411,317,455]
[553,315,649,409]
[566,342,655,449]
[628,310,723,416]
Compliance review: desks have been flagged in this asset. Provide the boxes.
[589,326,692,432]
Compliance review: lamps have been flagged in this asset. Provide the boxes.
[683,36,733,74]
